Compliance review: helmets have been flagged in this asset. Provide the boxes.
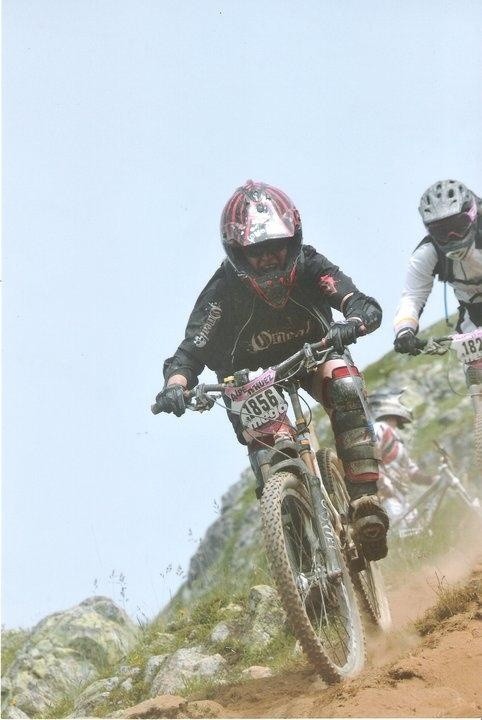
[418,179,477,260]
[365,383,413,423]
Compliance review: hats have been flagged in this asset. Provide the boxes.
[219,179,303,309]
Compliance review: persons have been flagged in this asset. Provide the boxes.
[393,181,481,416]
[156,182,391,625]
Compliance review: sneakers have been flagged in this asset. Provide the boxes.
[351,492,390,561]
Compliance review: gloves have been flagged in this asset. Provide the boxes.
[330,321,358,355]
[393,327,423,355]
[156,385,186,416]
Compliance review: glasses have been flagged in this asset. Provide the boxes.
[243,241,289,258]
